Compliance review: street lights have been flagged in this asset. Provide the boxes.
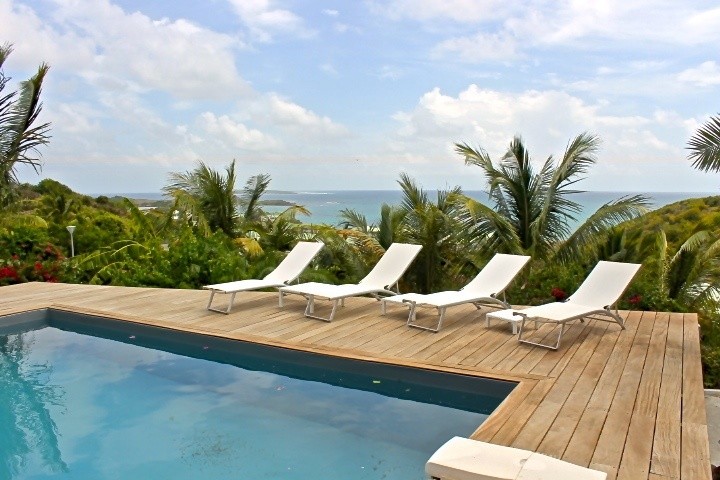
[66,226,76,258]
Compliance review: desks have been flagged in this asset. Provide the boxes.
[383,293,444,320]
[279,281,345,312]
[487,309,538,335]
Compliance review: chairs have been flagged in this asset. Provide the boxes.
[202,241,326,315]
[296,242,424,322]
[401,253,531,332]
[513,260,644,351]
